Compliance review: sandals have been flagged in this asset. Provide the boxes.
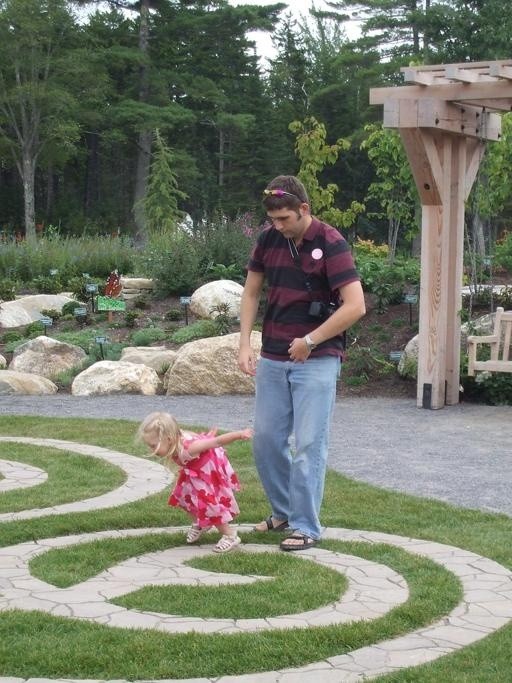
[212,530,241,552]
[253,514,289,532]
[186,518,204,543]
[280,534,317,549]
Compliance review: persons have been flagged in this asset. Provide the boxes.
[236,173,368,550]
[138,410,256,553]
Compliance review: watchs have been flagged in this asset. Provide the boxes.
[304,334,317,351]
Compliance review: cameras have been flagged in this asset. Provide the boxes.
[307,301,338,322]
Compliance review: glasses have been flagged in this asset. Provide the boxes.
[263,188,304,202]
